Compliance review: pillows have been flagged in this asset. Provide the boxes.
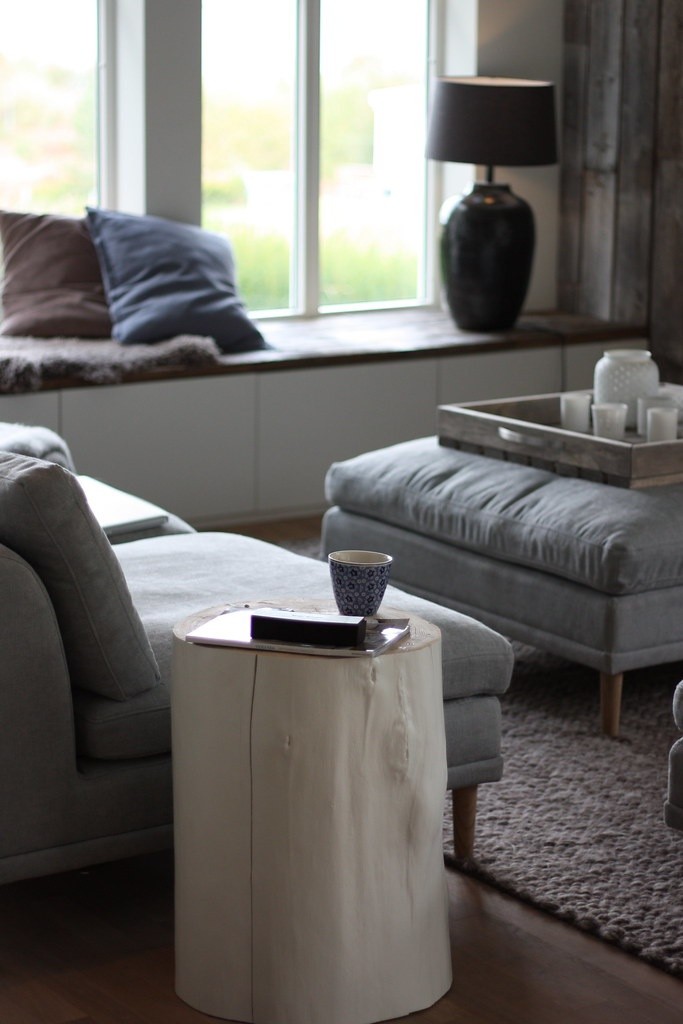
[0,209,113,340]
[84,205,268,351]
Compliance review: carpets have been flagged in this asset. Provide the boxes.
[263,533,683,983]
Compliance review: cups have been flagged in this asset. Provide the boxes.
[328,550,393,630]
[559,394,678,443]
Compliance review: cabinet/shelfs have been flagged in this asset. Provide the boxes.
[0,308,652,526]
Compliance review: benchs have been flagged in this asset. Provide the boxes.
[321,435,682,737]
[1,421,513,885]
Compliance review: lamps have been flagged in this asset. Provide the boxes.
[424,75,560,334]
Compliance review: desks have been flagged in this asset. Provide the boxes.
[171,599,453,1024]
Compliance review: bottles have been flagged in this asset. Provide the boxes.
[593,348,660,429]
